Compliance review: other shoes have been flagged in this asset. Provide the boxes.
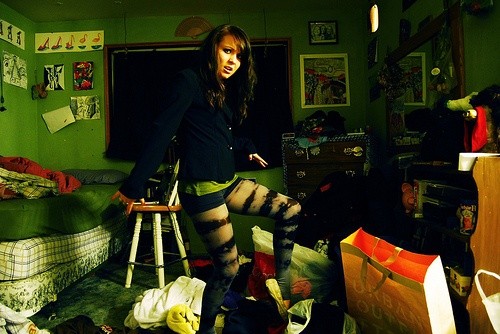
[265,278,288,321]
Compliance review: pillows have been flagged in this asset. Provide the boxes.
[56,168,128,183]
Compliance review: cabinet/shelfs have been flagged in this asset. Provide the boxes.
[282,130,371,235]
[400,92,500,334]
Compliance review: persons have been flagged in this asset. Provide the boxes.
[111,24,301,334]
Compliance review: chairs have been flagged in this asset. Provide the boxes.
[124,155,191,288]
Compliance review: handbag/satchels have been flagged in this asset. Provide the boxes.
[249,224,340,302]
[474,269,500,334]
[285,299,360,334]
[341,228,456,334]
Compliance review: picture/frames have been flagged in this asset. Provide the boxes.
[397,51,426,107]
[308,20,339,45]
[299,52,351,109]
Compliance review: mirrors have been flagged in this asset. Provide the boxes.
[386,8,466,163]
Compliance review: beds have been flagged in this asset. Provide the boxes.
[0,170,130,323]
